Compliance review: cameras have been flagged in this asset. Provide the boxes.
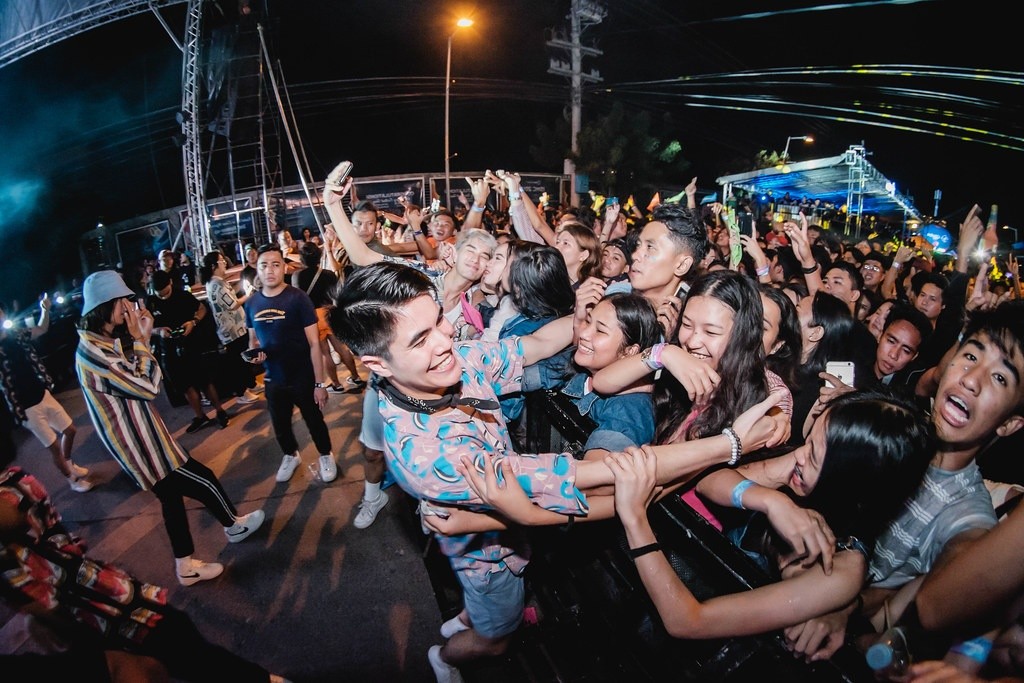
[170,328,186,356]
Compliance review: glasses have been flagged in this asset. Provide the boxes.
[863,263,884,273]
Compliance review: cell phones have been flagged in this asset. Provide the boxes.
[825,362,855,388]
[243,347,263,360]
[668,281,691,313]
[337,161,354,186]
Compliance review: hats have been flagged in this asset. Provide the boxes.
[81,270,137,317]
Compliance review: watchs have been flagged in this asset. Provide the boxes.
[642,347,656,371]
[314,383,325,388]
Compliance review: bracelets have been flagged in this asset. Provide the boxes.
[732,479,756,510]
[800,261,818,274]
[471,204,486,213]
[629,543,664,559]
[755,264,769,276]
[948,636,992,664]
[891,261,901,269]
[413,230,423,236]
[651,339,669,368]
[192,318,200,326]
[506,187,525,201]
[721,428,742,465]
[134,335,143,341]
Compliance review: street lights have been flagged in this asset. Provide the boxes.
[783,135,813,164]
[444,17,475,211]
[1003,225,1017,244]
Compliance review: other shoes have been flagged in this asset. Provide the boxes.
[325,383,345,394]
[346,375,363,387]
[439,614,469,639]
[429,646,463,683]
[248,382,265,394]
[237,391,258,405]
[200,397,210,405]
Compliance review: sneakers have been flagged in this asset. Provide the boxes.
[275,450,301,482]
[214,410,229,430]
[65,458,88,477]
[318,452,336,482]
[71,477,93,493]
[186,414,210,433]
[354,491,389,530]
[224,510,265,543]
[176,562,224,587]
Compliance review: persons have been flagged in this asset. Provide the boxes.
[0,171,1024,683]
